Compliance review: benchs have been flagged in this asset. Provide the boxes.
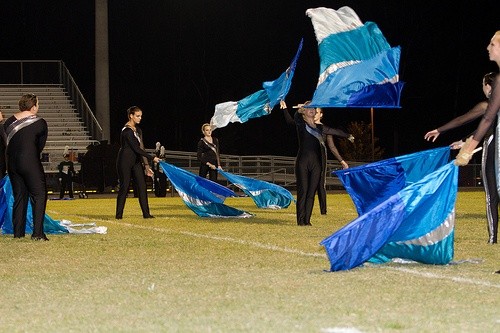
[0,84,102,178]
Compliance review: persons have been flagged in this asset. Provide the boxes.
[312,107,349,214]
[425,30,500,274]
[197,123,222,182]
[0,93,48,240]
[57,153,75,198]
[116,106,161,219]
[279,101,355,224]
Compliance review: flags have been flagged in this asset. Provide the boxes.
[209,35,303,128]
[321,147,458,273]
[0,174,108,234]
[157,159,297,219]
[306,6,406,107]
